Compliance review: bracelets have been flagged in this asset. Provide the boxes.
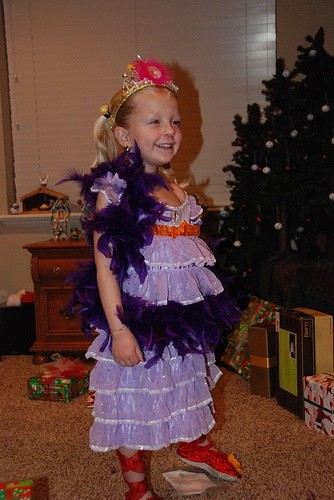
[110,325,129,335]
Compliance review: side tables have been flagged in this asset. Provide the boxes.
[22,236,102,365]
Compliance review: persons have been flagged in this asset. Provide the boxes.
[84,56,244,500]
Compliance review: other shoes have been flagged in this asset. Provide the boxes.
[177,441,242,483]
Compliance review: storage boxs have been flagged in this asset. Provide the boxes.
[27,353,97,402]
[219,292,284,384]
[302,372,334,437]
[273,306,334,420]
[247,321,280,398]
